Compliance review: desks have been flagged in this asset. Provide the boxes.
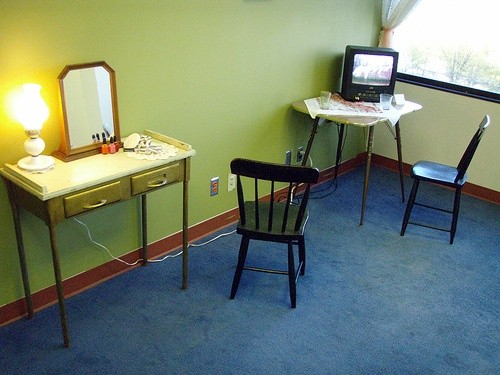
[291,92,423,225]
[2,128,197,348]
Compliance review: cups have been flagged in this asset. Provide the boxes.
[380,94,393,110]
[320,91,331,110]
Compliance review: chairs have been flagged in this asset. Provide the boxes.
[230,157,321,309]
[400,115,490,245]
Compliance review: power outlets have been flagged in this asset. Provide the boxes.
[285,146,304,165]
[210,177,219,196]
[227,174,237,192]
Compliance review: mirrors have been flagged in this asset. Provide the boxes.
[56,61,122,163]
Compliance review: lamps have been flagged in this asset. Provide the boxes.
[6,83,57,172]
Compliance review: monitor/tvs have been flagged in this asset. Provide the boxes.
[338,45,399,103]
